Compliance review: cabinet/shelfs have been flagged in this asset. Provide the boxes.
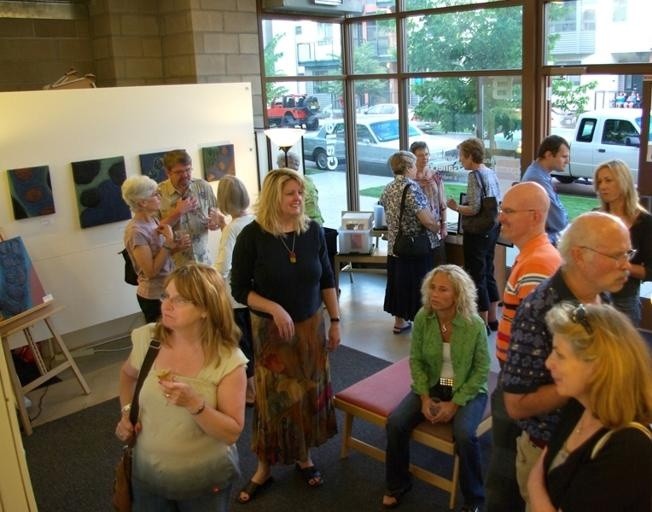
[444,232,507,308]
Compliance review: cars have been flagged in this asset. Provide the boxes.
[295,99,466,182]
[480,107,577,158]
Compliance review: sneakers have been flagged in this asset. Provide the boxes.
[383,479,414,506]
[393,321,412,334]
[484,318,500,334]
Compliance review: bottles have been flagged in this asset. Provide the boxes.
[373,205,384,229]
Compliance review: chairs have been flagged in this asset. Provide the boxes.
[323,226,341,296]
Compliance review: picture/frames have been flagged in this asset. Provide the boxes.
[457,192,513,248]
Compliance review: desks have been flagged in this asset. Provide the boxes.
[0,301,92,436]
[334,237,389,305]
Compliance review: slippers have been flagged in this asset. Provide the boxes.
[236,475,274,502]
[295,462,324,487]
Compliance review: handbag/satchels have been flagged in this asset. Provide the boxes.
[459,170,499,239]
[394,226,434,261]
[118,246,138,285]
[110,446,131,509]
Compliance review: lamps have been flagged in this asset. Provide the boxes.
[263,127,306,172]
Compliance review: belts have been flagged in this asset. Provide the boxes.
[439,377,454,389]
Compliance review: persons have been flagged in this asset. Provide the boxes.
[615,90,641,108]
[117,135,340,510]
[377,136,652,511]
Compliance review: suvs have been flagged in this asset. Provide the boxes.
[268,93,320,130]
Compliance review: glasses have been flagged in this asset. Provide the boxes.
[562,292,594,335]
[157,291,195,305]
[576,244,639,265]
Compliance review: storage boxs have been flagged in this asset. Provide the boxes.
[336,223,376,255]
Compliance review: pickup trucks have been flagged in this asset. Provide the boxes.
[548,106,652,188]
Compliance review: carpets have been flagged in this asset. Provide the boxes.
[20,338,496,512]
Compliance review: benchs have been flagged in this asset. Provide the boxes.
[329,349,504,510]
